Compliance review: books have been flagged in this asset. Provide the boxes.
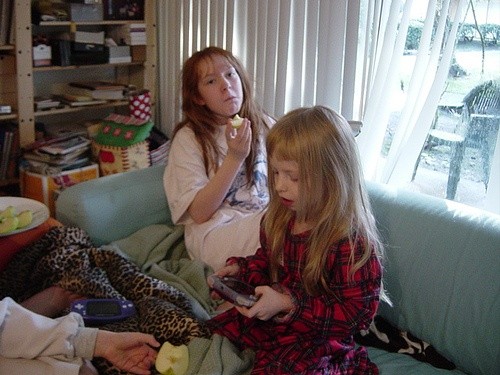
[0,81,130,181]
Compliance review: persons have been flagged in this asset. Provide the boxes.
[203,106,394,375]
[163,46,279,274]
[0,296,160,375]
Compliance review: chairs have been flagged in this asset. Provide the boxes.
[411,77,499,201]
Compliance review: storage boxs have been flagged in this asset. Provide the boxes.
[50,39,109,67]
[20,161,99,216]
[91,141,150,178]
[102,1,145,20]
[71,0,105,21]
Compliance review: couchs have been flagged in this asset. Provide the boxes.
[56,164,499,375]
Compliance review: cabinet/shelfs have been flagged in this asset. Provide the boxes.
[0,0,157,187]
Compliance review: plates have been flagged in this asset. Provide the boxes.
[0,196,49,235]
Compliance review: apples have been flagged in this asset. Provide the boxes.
[155,341,189,375]
[0,206,33,233]
[230,114,243,128]
